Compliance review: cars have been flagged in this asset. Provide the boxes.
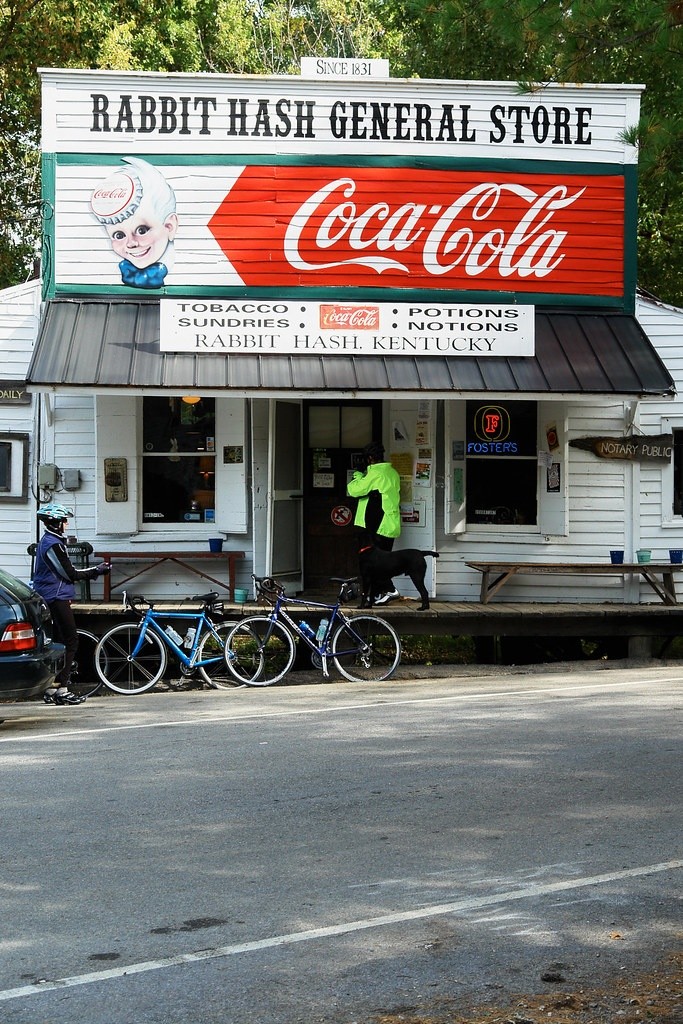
[0,568,66,699]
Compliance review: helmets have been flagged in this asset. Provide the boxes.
[365,441,386,454]
[37,504,74,521]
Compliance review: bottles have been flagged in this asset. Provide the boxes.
[164,624,184,646]
[298,621,316,638]
[184,625,197,649]
[315,618,331,641]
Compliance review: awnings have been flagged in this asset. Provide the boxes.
[24,292,675,424]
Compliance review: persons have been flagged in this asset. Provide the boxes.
[348,442,403,604]
[33,504,111,705]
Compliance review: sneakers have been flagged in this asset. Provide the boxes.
[44,691,60,704]
[366,593,391,605]
[386,590,400,600]
[54,692,86,705]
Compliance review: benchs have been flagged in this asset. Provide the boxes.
[95,548,246,602]
[466,559,682,607]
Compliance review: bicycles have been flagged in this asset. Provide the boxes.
[71,574,401,697]
